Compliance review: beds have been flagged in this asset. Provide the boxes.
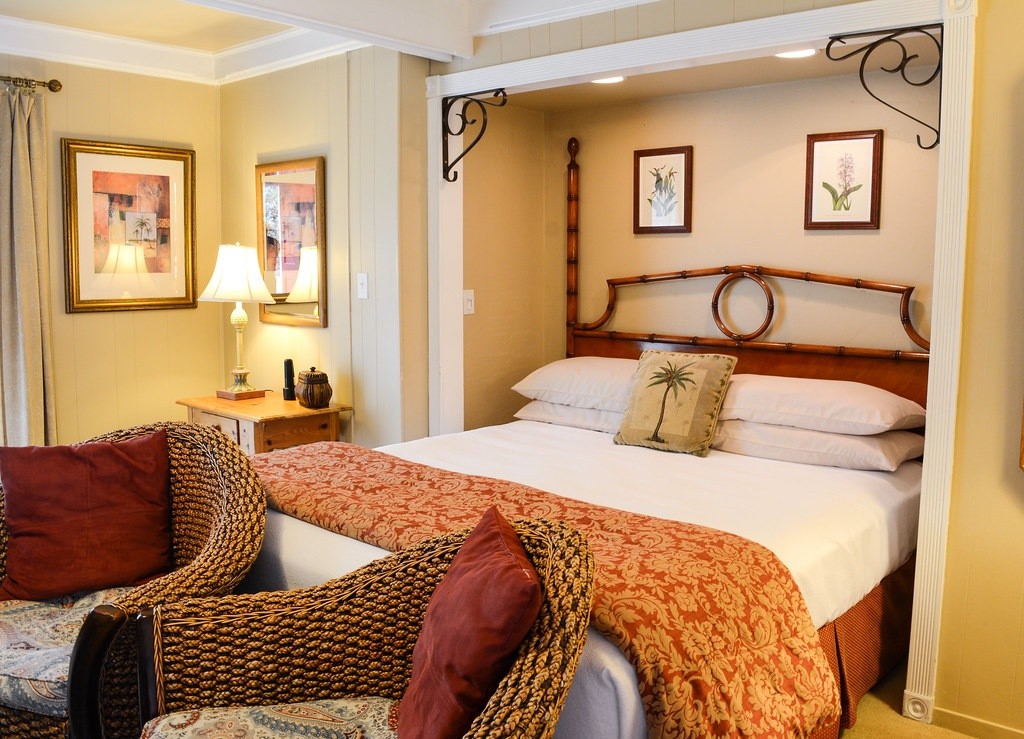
[235,137,938,738]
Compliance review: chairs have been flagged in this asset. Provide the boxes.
[0,417,266,739]
[133,518,594,739]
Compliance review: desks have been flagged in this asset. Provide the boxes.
[175,393,355,452]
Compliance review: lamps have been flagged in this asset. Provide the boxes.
[195,241,277,400]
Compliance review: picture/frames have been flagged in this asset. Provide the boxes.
[634,145,692,234]
[805,130,883,229]
[57,139,196,314]
[254,156,327,328]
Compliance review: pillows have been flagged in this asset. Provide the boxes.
[510,354,640,414]
[395,505,542,738]
[611,350,738,456]
[709,420,925,472]
[716,373,925,436]
[512,401,628,433]
[0,427,173,601]
